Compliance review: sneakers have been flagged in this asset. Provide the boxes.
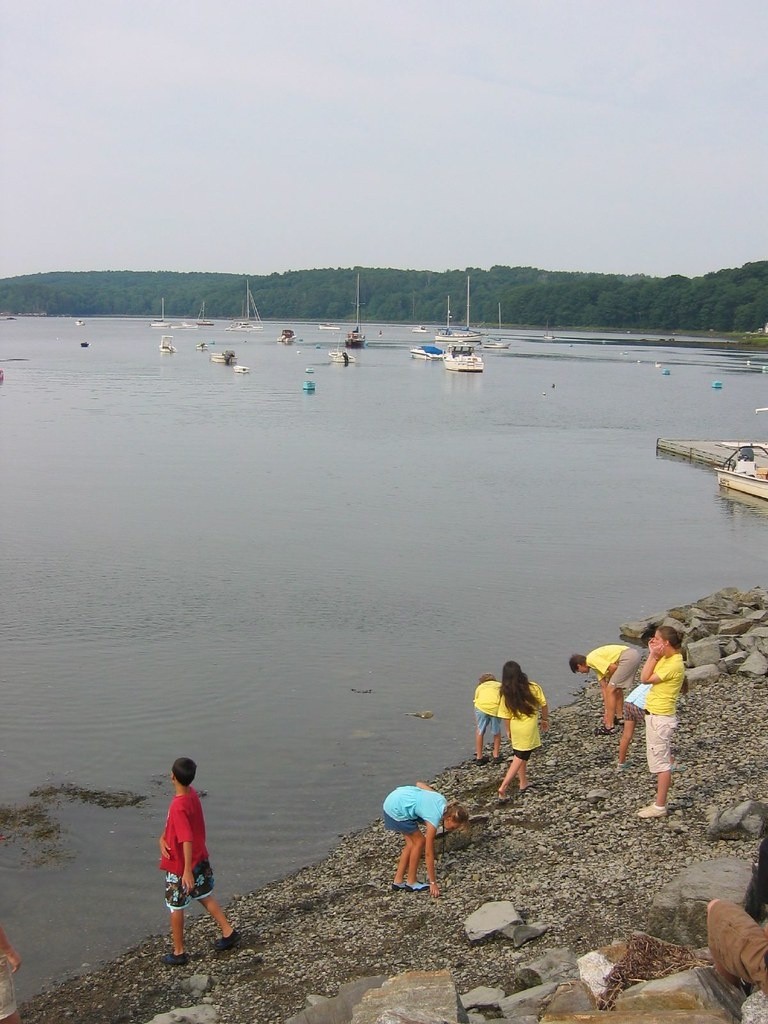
[214,929,242,949]
[404,880,430,891]
[494,755,503,764]
[161,953,187,965]
[638,802,667,818]
[392,881,405,890]
[476,755,489,766]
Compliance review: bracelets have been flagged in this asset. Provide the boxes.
[542,718,547,721]
[429,881,436,885]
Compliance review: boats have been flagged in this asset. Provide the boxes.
[210,349,238,364]
[158,335,178,353]
[197,343,208,350]
[233,364,251,374]
[75,319,86,326]
[319,323,341,330]
[328,333,356,363]
[443,342,484,372]
[412,325,431,333]
[170,321,199,330]
[409,345,446,361]
[276,330,297,343]
[714,442,768,501]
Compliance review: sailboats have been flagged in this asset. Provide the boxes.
[435,275,485,342]
[344,273,366,348]
[544,319,555,339]
[483,302,511,349]
[149,298,168,327]
[225,279,264,331]
[196,302,215,325]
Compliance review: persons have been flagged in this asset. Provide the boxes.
[157,756,240,965]
[616,674,689,772]
[707,838,768,999]
[552,383,555,389]
[497,660,548,801]
[0,923,23,1024]
[383,781,470,898]
[641,629,656,646]
[637,626,685,819]
[472,671,503,766]
[569,644,642,735]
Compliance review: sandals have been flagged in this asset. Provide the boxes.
[613,717,625,725]
[618,760,633,772]
[595,725,616,736]
[671,761,688,773]
